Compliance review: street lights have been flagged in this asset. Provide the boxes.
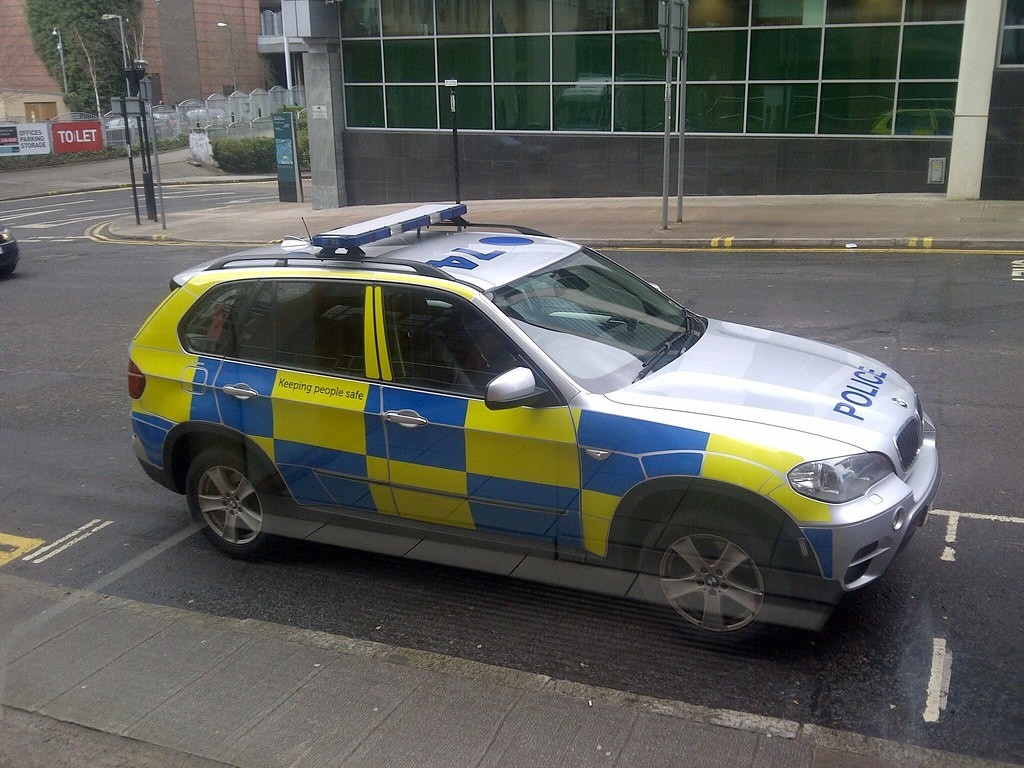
[51,27,69,93]
[99,13,131,98]
[216,22,238,94]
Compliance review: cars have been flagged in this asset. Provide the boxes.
[0,222,20,277]
[551,73,954,139]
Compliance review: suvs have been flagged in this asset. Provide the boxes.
[125,201,941,651]
